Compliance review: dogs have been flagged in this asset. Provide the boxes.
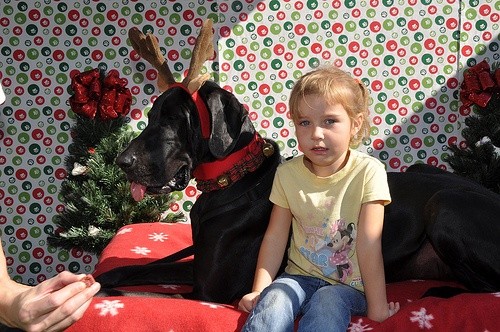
[115,81,500,305]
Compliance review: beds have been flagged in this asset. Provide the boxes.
[66,222,500,332]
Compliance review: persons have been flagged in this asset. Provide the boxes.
[0,79,101,332]
[238,68,399,332]
